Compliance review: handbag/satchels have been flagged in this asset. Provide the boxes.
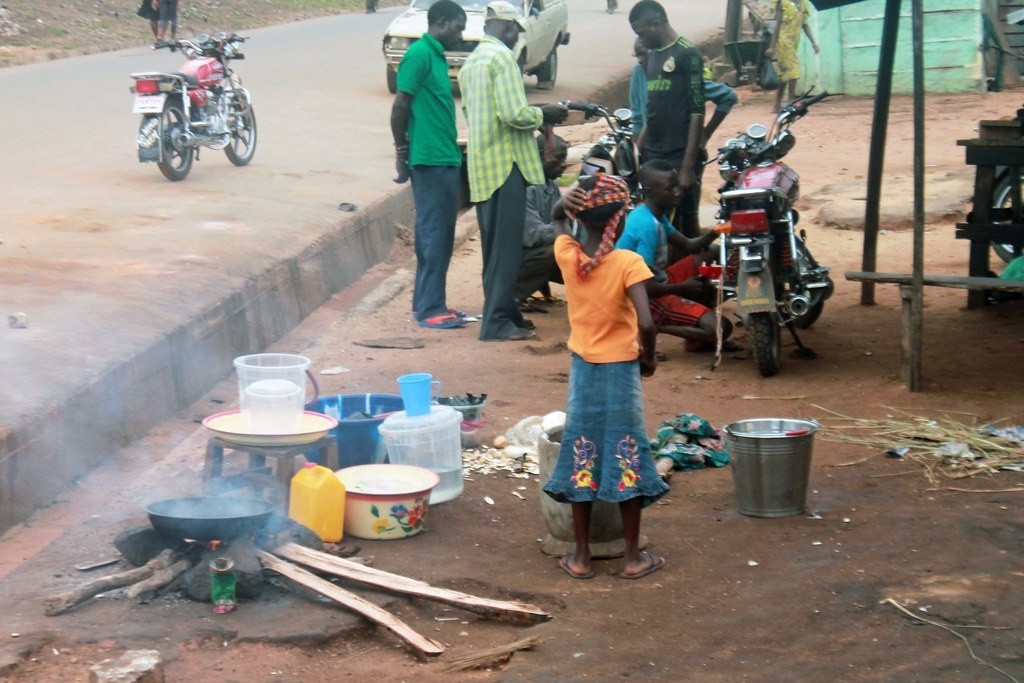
[757,56,782,91]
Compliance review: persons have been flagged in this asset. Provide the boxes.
[542,170,665,580]
[365,0,379,14]
[605,0,618,14]
[628,0,736,246]
[389,0,463,329]
[456,1,573,342]
[614,160,738,350]
[137,0,178,49]
[765,0,820,113]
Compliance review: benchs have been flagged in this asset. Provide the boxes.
[845,270,1024,388]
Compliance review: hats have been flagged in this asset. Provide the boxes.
[483,1,526,33]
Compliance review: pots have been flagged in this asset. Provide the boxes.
[144,496,274,541]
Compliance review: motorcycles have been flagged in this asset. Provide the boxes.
[557,98,639,245]
[128,31,258,183]
[969,101,1024,265]
[700,84,845,378]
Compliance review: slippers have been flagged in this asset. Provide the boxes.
[515,318,535,329]
[654,351,667,361]
[559,553,595,579]
[414,309,467,329]
[480,327,537,340]
[722,340,744,352]
[620,551,666,579]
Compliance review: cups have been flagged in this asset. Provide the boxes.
[397,373,441,416]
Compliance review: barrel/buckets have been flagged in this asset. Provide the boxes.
[302,394,405,472]
[289,462,346,543]
[722,418,820,517]
[377,405,464,505]
[234,353,320,434]
[438,397,490,448]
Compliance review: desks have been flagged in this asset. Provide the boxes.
[957,135,1024,311]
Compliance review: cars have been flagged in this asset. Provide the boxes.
[382,0,572,95]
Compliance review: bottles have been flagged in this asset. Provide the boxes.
[655,457,673,475]
[209,557,237,614]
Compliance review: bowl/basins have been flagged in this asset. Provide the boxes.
[246,380,302,422]
[334,464,442,539]
